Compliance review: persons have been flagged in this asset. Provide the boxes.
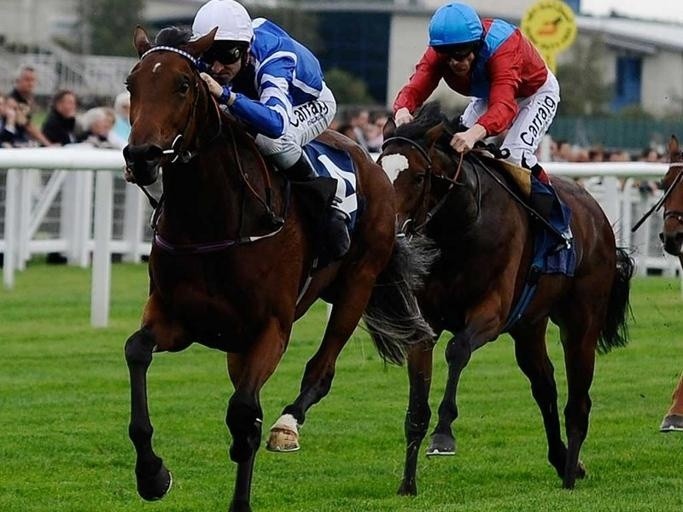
[191,0,356,260]
[391,1,571,253]
[0,65,134,265]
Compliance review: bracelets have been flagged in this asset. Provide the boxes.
[218,84,232,104]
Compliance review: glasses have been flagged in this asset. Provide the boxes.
[439,49,470,62]
[202,46,242,64]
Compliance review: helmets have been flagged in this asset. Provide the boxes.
[427,2,482,46]
[188,0,253,43]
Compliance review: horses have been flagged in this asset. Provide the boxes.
[122,24,442,512]
[658,134,683,432]
[376,99,645,495]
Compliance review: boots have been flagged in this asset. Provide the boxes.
[284,149,350,256]
